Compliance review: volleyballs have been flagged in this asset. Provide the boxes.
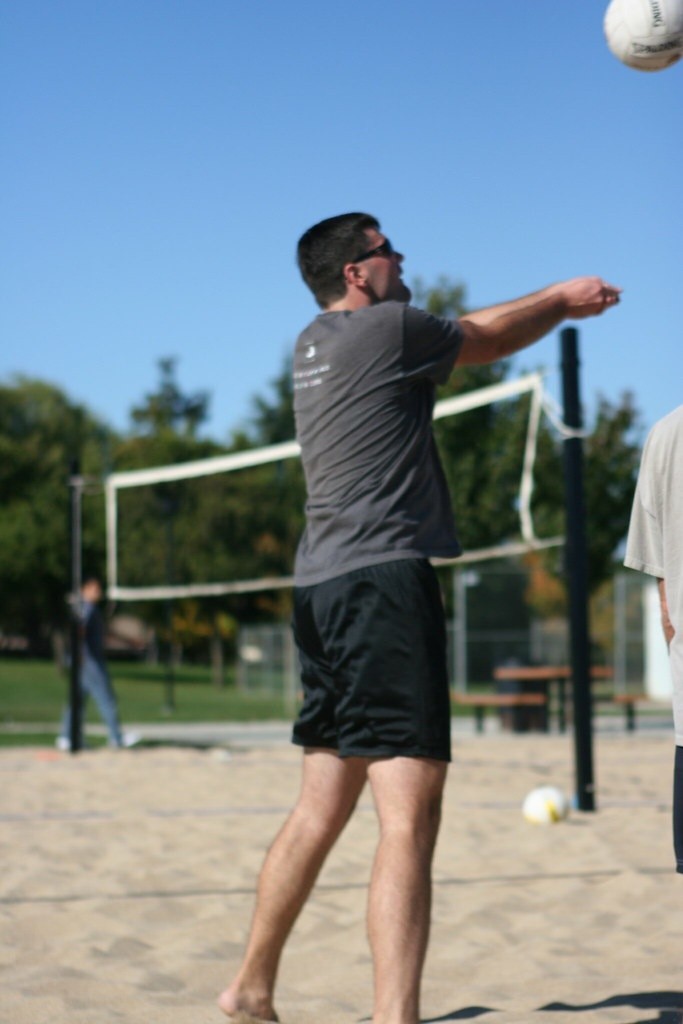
[522,788,567,824]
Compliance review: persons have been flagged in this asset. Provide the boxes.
[618,399,680,871]
[219,207,626,1024]
[50,577,148,750]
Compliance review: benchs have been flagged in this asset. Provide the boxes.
[551,693,649,732]
[451,693,545,733]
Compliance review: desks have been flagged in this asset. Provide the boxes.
[494,664,613,734]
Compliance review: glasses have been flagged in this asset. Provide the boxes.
[335,239,393,281]
